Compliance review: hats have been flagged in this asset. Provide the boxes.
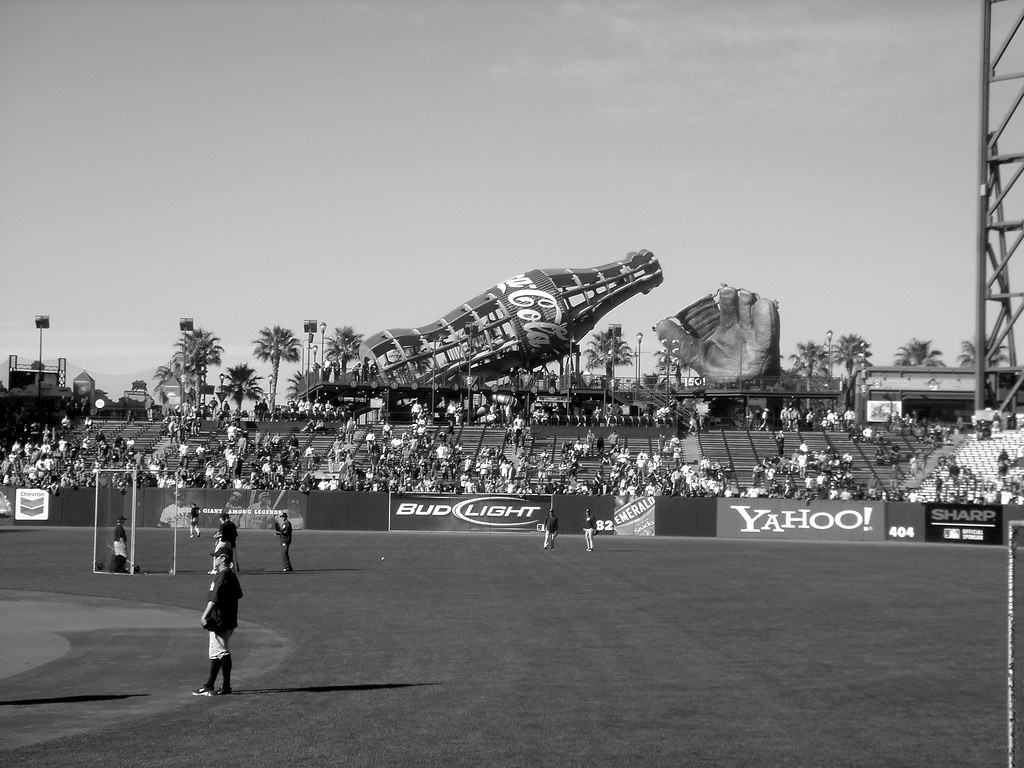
[764,408,769,411]
[280,513,287,518]
[369,430,372,432]
[367,468,371,471]
[255,429,259,431]
[586,509,590,512]
[549,509,553,512]
[774,454,777,456]
[821,450,825,453]
[783,454,786,456]
[827,410,831,413]
[311,475,315,478]
[226,514,229,517]
[209,546,234,560]
[789,408,792,411]
[218,514,226,518]
[197,417,200,420]
[61,436,64,438]
[45,424,48,427]
[981,421,984,424]
[117,516,126,520]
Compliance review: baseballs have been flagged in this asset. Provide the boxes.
[380,557,385,561]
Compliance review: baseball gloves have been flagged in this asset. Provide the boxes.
[275,522,281,532]
[655,282,782,383]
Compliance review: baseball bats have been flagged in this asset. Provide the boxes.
[108,545,131,563]
[544,534,556,548]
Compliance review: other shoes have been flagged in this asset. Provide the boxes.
[208,570,218,575]
[587,549,593,551]
[190,535,194,538]
[198,532,200,537]
[283,568,293,571]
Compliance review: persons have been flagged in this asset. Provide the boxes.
[543,508,559,550]
[273,512,292,573]
[0,369,1024,518]
[190,546,244,697]
[207,511,238,575]
[112,515,128,574]
[188,502,201,539]
[157,489,194,529]
[219,492,246,529]
[583,508,597,552]
[239,492,276,530]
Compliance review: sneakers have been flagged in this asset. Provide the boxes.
[191,686,216,697]
[215,686,233,695]
